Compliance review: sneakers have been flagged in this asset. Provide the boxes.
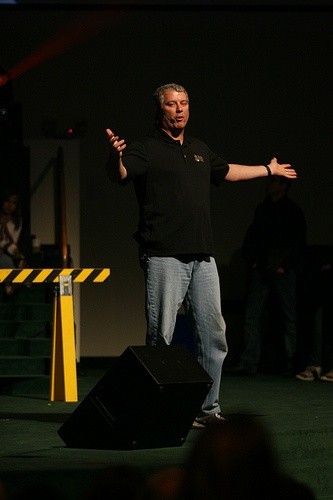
[192,414,232,430]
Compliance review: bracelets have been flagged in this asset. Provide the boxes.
[262,163,272,177]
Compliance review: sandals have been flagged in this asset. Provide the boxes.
[296,367,322,381]
[320,370,333,382]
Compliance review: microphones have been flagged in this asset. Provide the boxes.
[166,119,175,123]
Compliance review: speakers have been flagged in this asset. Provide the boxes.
[58,345,214,451]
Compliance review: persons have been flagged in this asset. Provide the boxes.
[223,176,307,374]
[0,189,22,268]
[296,306,333,382]
[104,83,297,428]
[0,417,317,500]
